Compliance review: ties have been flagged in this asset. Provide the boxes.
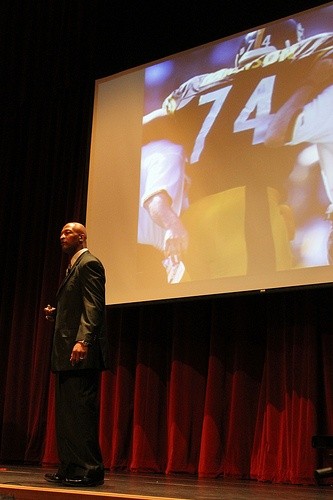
[66,264,72,276]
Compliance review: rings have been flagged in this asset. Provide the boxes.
[80,358,83,359]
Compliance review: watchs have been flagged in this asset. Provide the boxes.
[78,340,89,346]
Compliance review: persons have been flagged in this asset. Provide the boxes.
[139,17,333,284]
[44,222,105,487]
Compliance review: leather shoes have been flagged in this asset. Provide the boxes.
[66,469,105,488]
[43,471,66,484]
